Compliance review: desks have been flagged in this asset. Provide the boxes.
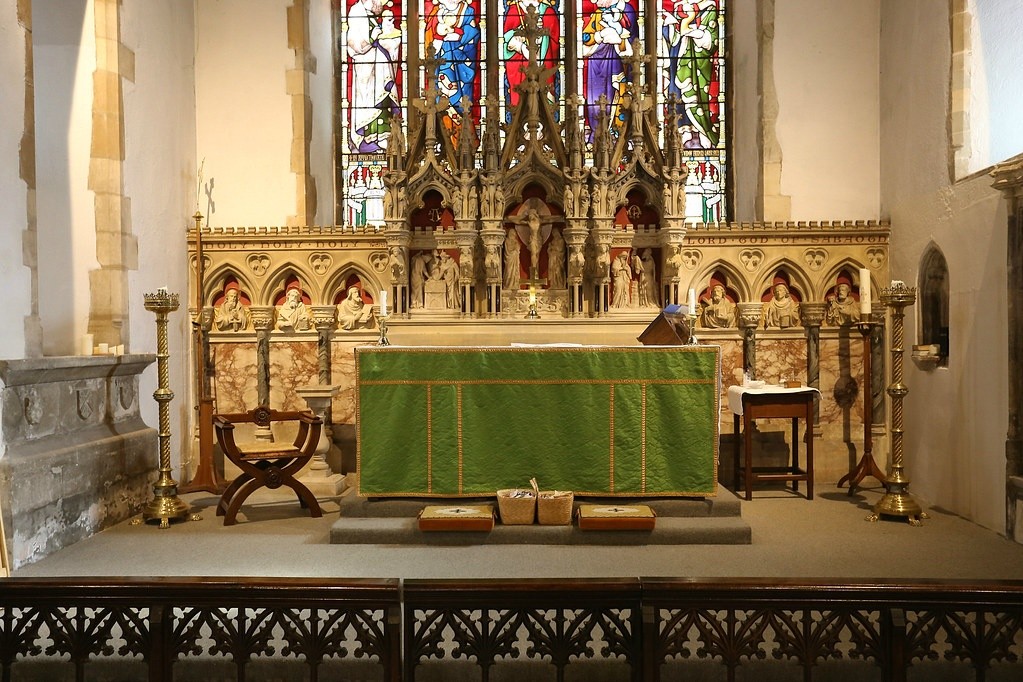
[353,346,723,502]
[727,383,825,501]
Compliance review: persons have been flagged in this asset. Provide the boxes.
[611,251,632,307]
[639,249,660,307]
[764,284,799,327]
[410,249,460,309]
[451,185,505,218]
[563,184,617,218]
[827,284,861,326]
[504,209,567,290]
[660,183,686,215]
[383,186,408,219]
[526,74,539,116]
[338,288,373,330]
[215,289,248,331]
[526,4,539,32]
[277,289,308,330]
[702,285,736,328]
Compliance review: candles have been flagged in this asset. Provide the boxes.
[859,264,872,314]
[688,288,696,315]
[81,332,124,356]
[380,289,386,316]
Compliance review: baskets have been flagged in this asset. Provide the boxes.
[534,478,574,525]
[496,480,537,525]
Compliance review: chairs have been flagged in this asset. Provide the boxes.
[212,405,325,526]
